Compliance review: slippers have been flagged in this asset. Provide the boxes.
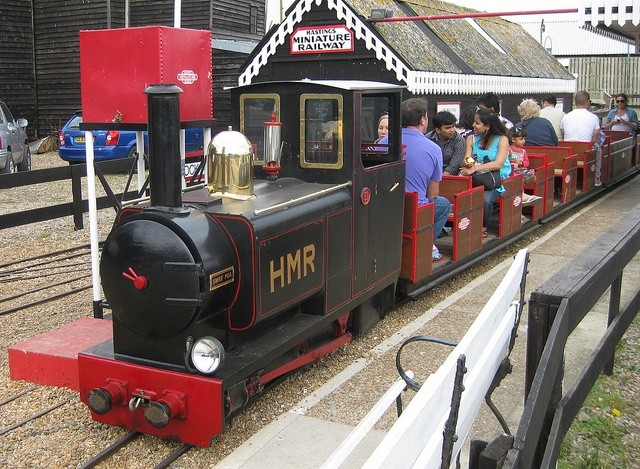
[521,195,543,207]
[482,230,488,238]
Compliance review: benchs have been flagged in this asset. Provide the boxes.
[557,141,597,192]
[602,130,629,141]
[601,136,611,183]
[523,153,556,220]
[522,146,578,203]
[399,191,436,284]
[494,164,524,237]
[440,175,485,262]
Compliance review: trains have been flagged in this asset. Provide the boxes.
[77,0,640,449]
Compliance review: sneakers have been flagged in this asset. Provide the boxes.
[594,177,602,186]
[436,228,449,239]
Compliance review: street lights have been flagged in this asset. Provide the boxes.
[540,18,546,45]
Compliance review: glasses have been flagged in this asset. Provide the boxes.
[616,99,626,104]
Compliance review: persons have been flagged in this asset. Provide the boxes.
[561,91,602,187]
[507,125,529,174]
[375,97,450,261]
[606,93,638,139]
[455,106,477,140]
[424,112,466,177]
[459,110,510,237]
[539,93,565,142]
[365,114,389,153]
[477,92,512,128]
[517,99,558,147]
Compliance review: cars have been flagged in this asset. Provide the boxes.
[0,101,32,175]
[58,110,215,174]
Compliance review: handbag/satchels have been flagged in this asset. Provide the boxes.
[459,156,501,190]
